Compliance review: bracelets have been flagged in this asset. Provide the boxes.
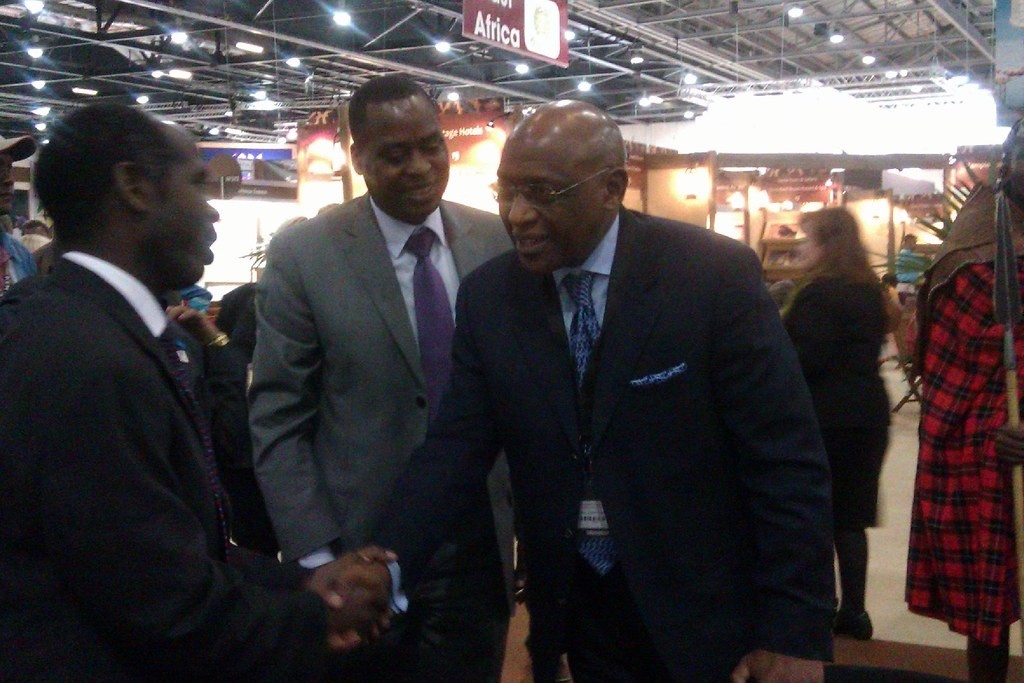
[207,331,230,347]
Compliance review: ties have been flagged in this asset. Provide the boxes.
[405,228,456,426]
[562,271,622,576]
[160,323,232,568]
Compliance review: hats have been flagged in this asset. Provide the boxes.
[0,131,37,163]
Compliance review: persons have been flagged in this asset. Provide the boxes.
[898,233,930,283]
[0,104,399,683]
[11,214,55,255]
[328,99,834,683]
[881,269,915,309]
[0,130,41,300]
[781,207,892,640]
[246,73,512,683]
[904,117,1024,683]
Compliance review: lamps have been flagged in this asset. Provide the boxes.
[830,27,844,44]
[630,51,644,64]
[639,90,651,107]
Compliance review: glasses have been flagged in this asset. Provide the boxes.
[489,168,610,206]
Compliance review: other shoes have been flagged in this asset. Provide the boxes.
[834,609,872,640]
